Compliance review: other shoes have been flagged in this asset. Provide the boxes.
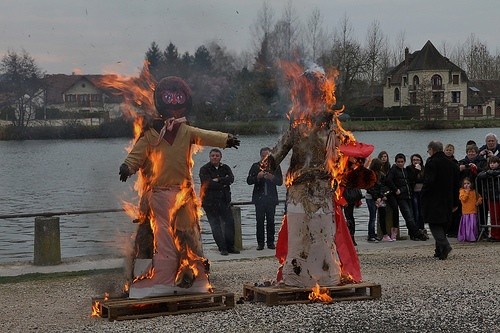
[409,229,430,241]
[352,238,357,245]
[447,231,457,238]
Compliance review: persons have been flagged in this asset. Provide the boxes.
[479,133,500,235]
[247,147,283,250]
[443,144,460,238]
[406,154,430,240]
[260,69,375,288]
[340,155,365,246]
[199,149,241,256]
[386,153,426,241]
[458,177,482,243]
[119,76,242,297]
[377,151,399,242]
[419,141,460,260]
[478,156,500,242]
[459,144,488,239]
[366,158,383,243]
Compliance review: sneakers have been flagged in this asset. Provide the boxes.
[221,247,240,255]
[434,244,453,259]
[368,236,380,243]
[255,243,275,250]
[382,234,397,241]
